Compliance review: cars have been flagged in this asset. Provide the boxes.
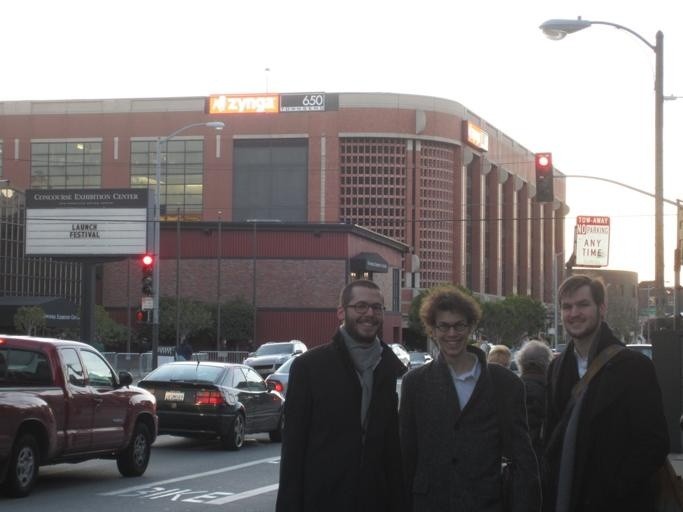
[242,334,431,402]
[140,344,176,372]
[139,353,285,455]
[506,341,653,369]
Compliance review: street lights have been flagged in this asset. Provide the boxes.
[0,178,17,199]
[537,12,667,329]
[150,120,226,371]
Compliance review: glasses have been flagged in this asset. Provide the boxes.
[432,323,472,332]
[346,302,386,314]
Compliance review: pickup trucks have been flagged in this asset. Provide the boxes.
[1,330,163,500]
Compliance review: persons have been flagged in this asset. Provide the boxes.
[488,343,512,369]
[539,275,670,512]
[397,283,544,512]
[512,339,553,456]
[273,279,412,511]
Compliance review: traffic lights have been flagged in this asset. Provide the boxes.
[142,253,153,298]
[135,309,153,324]
[533,151,554,205]
